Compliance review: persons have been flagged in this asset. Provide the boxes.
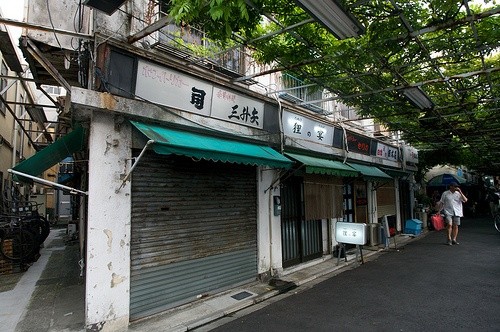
[434,181,500,220]
[436,181,468,246]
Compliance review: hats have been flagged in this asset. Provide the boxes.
[450,182,459,186]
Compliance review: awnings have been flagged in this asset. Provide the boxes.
[344,161,394,191]
[51,176,74,194]
[280,152,361,185]
[7,120,90,199]
[427,173,470,189]
[117,117,295,194]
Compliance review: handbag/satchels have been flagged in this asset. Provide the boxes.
[431,214,445,231]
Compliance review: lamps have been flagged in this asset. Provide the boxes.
[404,86,436,112]
[294,0,365,40]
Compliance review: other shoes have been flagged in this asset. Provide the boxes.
[452,239,459,244]
[447,239,452,245]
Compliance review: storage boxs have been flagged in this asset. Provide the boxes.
[405,219,423,234]
